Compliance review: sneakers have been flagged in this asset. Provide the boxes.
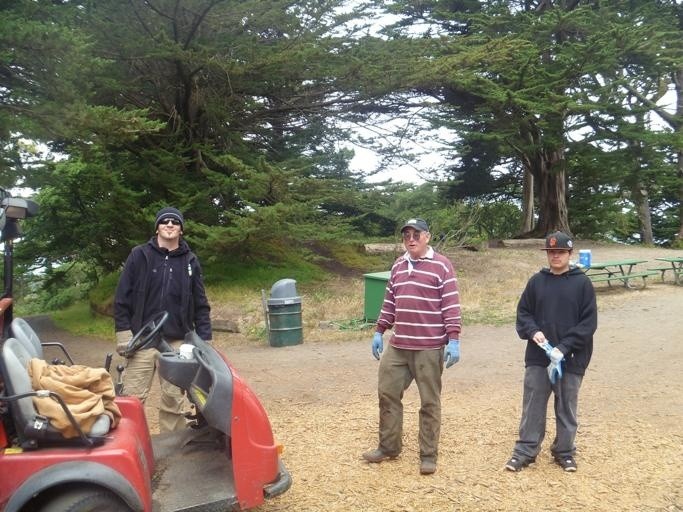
[362,447,402,463]
[552,450,578,473]
[505,454,537,473]
[420,455,437,475]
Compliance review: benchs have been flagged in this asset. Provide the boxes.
[567,257,682,292]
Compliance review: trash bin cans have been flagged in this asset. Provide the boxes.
[361,271,392,321]
[267,278,303,347]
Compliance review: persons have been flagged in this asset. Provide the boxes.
[506,231,598,472]
[363,219,463,474]
[113,207,212,434]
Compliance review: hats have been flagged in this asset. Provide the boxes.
[400,217,430,234]
[154,207,185,232]
[539,230,574,251]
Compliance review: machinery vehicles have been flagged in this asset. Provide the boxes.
[0,186,292,512]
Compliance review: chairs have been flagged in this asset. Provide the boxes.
[10,315,76,370]
[0,335,113,447]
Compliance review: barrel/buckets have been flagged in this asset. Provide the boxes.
[266,297,303,348]
[578,249,592,267]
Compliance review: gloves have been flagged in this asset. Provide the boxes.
[371,331,383,360]
[538,338,566,385]
[114,329,136,360]
[443,338,460,369]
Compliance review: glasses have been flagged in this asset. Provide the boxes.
[156,218,182,225]
[403,232,421,240]
